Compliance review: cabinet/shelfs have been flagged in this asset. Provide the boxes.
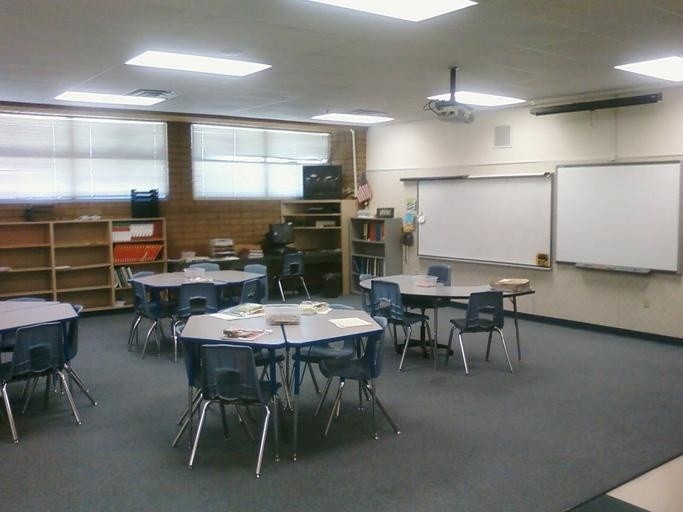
[0,217,168,313]
[351,216,402,294]
[280,199,357,294]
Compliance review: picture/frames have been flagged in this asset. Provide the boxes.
[377,207,394,218]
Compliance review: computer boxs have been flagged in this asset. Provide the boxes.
[273,247,297,255]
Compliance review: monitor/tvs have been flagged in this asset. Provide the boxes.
[303,165,343,199]
[269,224,295,248]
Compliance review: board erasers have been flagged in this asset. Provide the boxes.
[537,254,549,266]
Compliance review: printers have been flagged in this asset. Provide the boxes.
[209,238,236,259]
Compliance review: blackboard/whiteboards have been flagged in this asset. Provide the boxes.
[552,160,683,274]
[416,172,552,271]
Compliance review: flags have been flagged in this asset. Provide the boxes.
[356,174,372,202]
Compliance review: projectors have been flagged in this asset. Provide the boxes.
[433,105,474,123]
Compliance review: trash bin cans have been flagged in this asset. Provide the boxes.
[321,272,341,298]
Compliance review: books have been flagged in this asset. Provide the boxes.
[490,277,531,293]
[237,247,264,259]
[224,302,264,316]
[209,237,236,258]
[113,243,163,262]
[111,220,163,240]
[115,296,126,306]
[363,222,384,241]
[113,265,133,288]
[352,257,384,277]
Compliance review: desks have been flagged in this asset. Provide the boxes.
[359,275,535,369]
